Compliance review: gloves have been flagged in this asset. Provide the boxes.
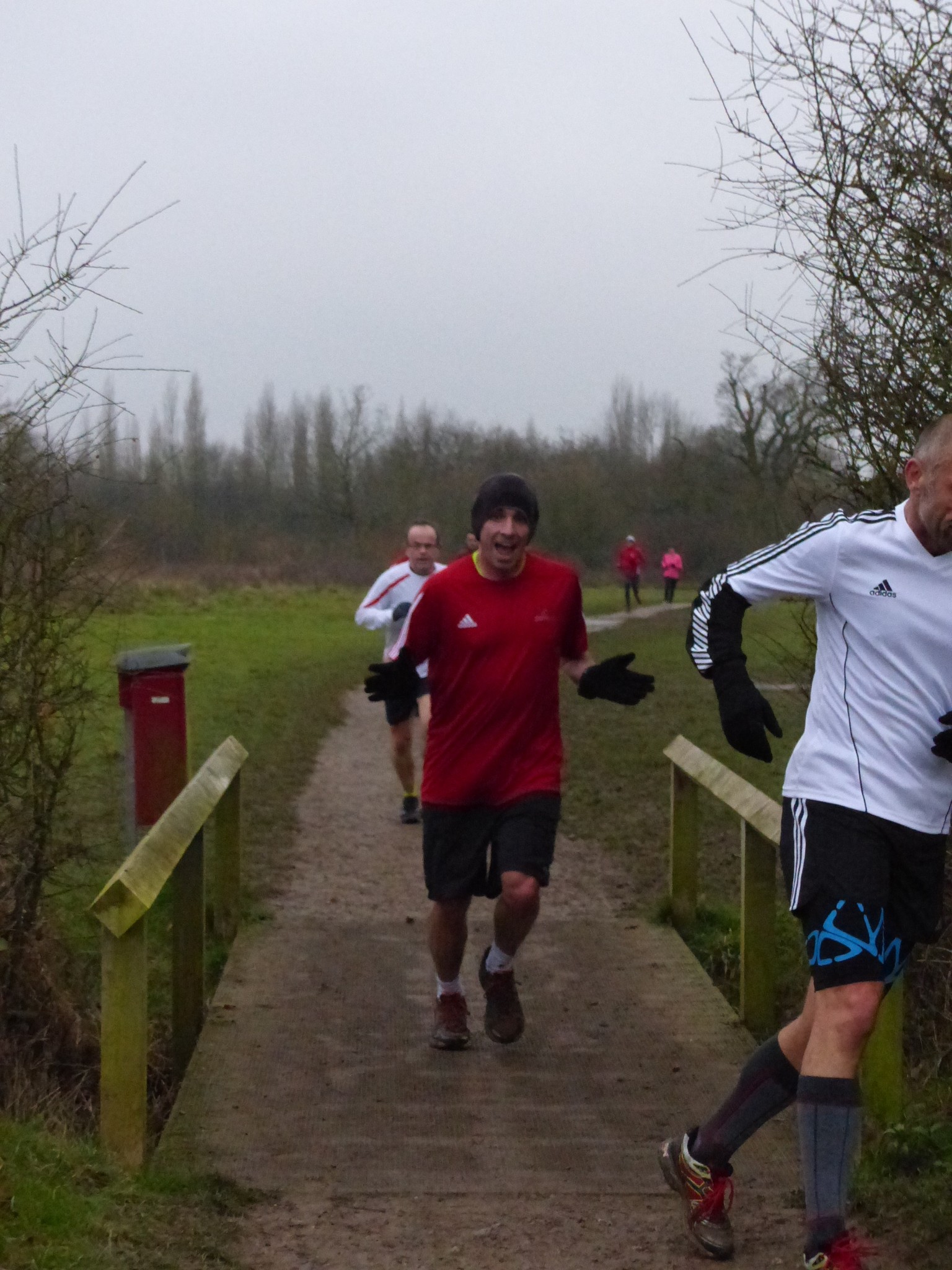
[931,710,952,763]
[578,651,656,705]
[363,663,422,702]
[712,666,783,764]
[393,602,412,622]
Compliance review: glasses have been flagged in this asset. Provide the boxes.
[407,542,437,549]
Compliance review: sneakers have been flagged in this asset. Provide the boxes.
[657,1125,736,1261]
[401,797,421,824]
[479,946,526,1043]
[430,994,471,1049]
[803,1229,868,1270]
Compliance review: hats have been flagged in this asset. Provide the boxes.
[626,535,636,543]
[471,474,539,543]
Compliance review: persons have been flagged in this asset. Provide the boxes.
[353,521,682,823]
[364,473,655,1046]
[657,413,952,1270]
